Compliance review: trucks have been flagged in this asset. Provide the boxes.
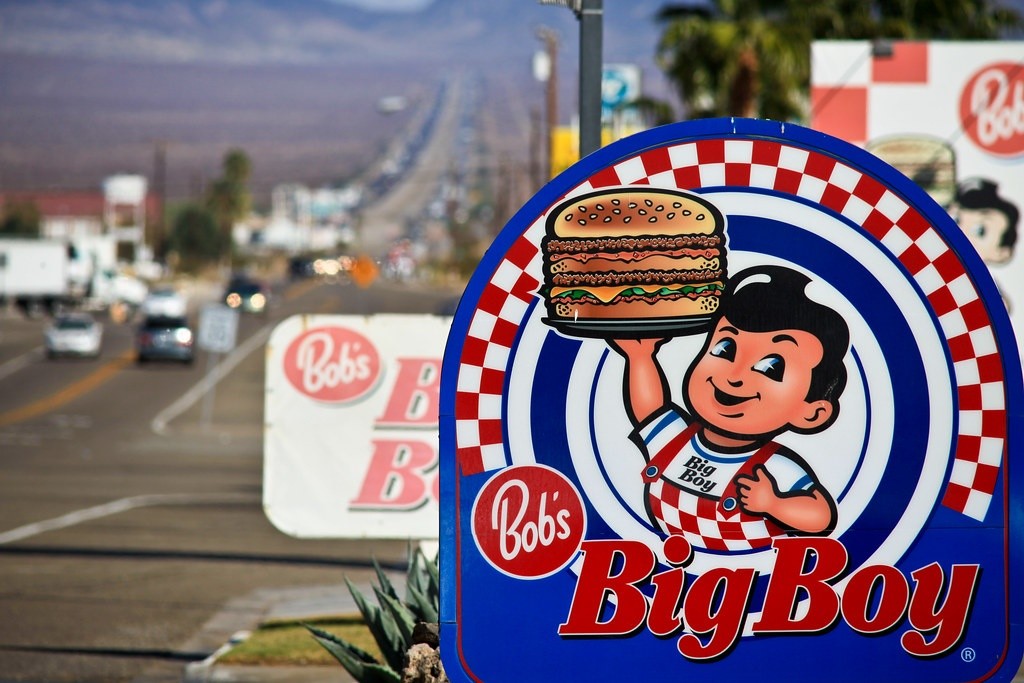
[0,236,150,320]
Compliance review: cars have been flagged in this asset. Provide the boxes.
[44,312,103,362]
[138,314,197,367]
[219,278,269,318]
